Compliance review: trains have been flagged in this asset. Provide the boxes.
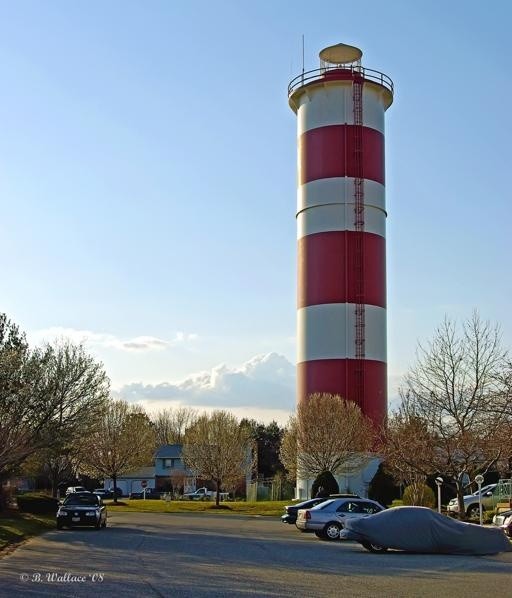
[141,479,147,488]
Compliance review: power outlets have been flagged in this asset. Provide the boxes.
[130,487,230,501]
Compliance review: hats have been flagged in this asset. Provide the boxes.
[281,493,388,554]
[447,482,512,537]
[56,486,122,530]
